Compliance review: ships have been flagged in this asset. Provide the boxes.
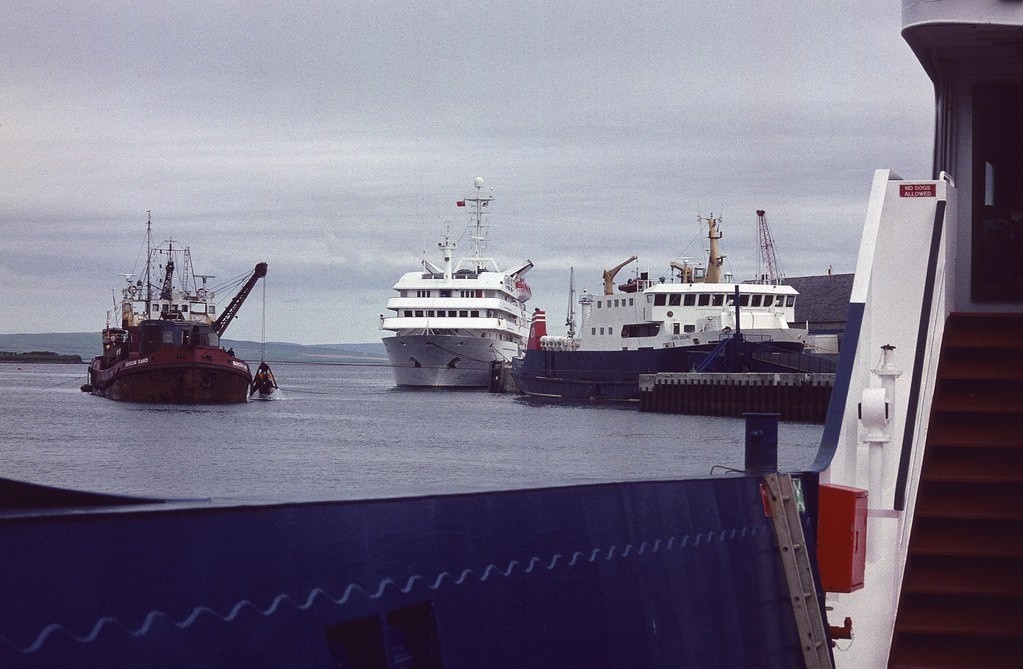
[377,177,533,392]
[511,204,839,416]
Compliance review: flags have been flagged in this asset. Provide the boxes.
[457,201,465,207]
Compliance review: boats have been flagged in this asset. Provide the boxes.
[79,208,270,406]
[516,281,532,301]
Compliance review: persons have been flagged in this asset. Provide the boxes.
[806,374,810,381]
[221,345,235,358]
